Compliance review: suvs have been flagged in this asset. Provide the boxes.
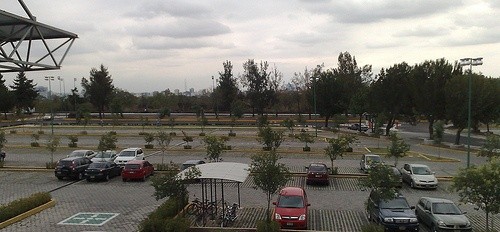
[114,147,146,168]
[53,156,93,180]
[366,188,420,232]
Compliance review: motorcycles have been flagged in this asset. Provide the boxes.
[222,202,240,227]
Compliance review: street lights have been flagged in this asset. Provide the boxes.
[459,57,483,170]
[309,76,320,124]
[45,75,55,133]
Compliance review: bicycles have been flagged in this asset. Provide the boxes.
[186,195,218,227]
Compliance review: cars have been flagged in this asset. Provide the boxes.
[121,160,155,181]
[359,153,385,173]
[415,196,473,232]
[67,149,119,164]
[304,161,331,185]
[84,161,121,182]
[369,163,403,187]
[348,122,369,131]
[179,159,206,170]
[272,187,311,230]
[399,164,439,190]
